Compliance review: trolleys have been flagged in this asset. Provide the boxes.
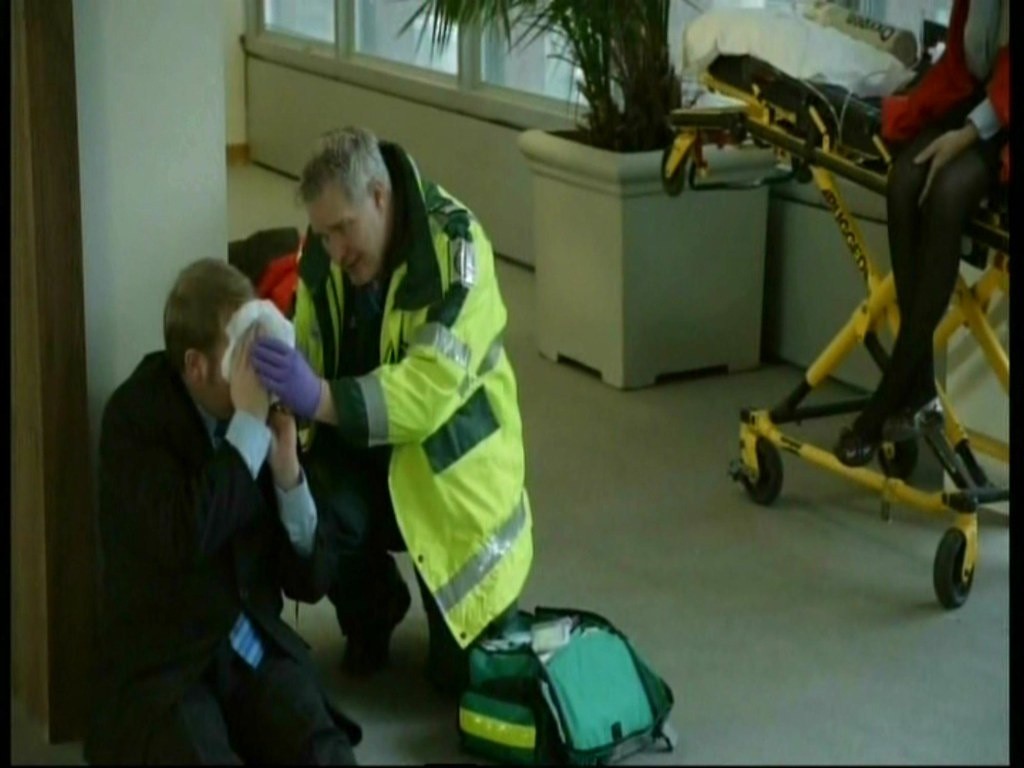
[662,57,1010,607]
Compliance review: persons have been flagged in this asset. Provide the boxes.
[249,124,534,705]
[90,257,364,768]
[835,0,1009,480]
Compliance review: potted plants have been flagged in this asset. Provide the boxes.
[395,0,780,384]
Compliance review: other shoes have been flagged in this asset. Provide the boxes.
[323,699,363,747]
[439,663,466,702]
[344,584,412,680]
[838,389,942,481]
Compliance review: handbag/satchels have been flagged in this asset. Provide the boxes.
[455,600,674,767]
